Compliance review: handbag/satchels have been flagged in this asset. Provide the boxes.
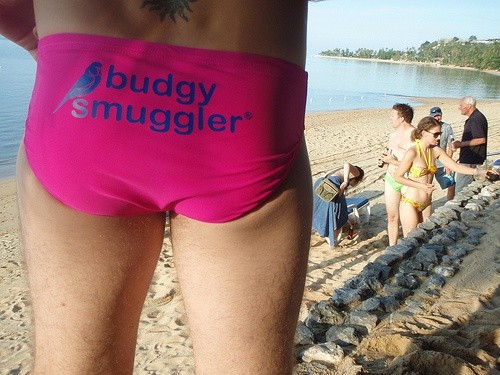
[313,178,339,204]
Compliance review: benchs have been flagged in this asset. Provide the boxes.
[336,191,384,243]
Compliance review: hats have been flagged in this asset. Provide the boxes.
[429,107,442,117]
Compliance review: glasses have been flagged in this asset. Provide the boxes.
[424,130,443,139]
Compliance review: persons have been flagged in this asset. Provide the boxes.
[0,1,317,375]
[393,116,500,238]
[452,96,489,196]
[428,106,456,202]
[313,162,364,250]
[377,102,418,246]
